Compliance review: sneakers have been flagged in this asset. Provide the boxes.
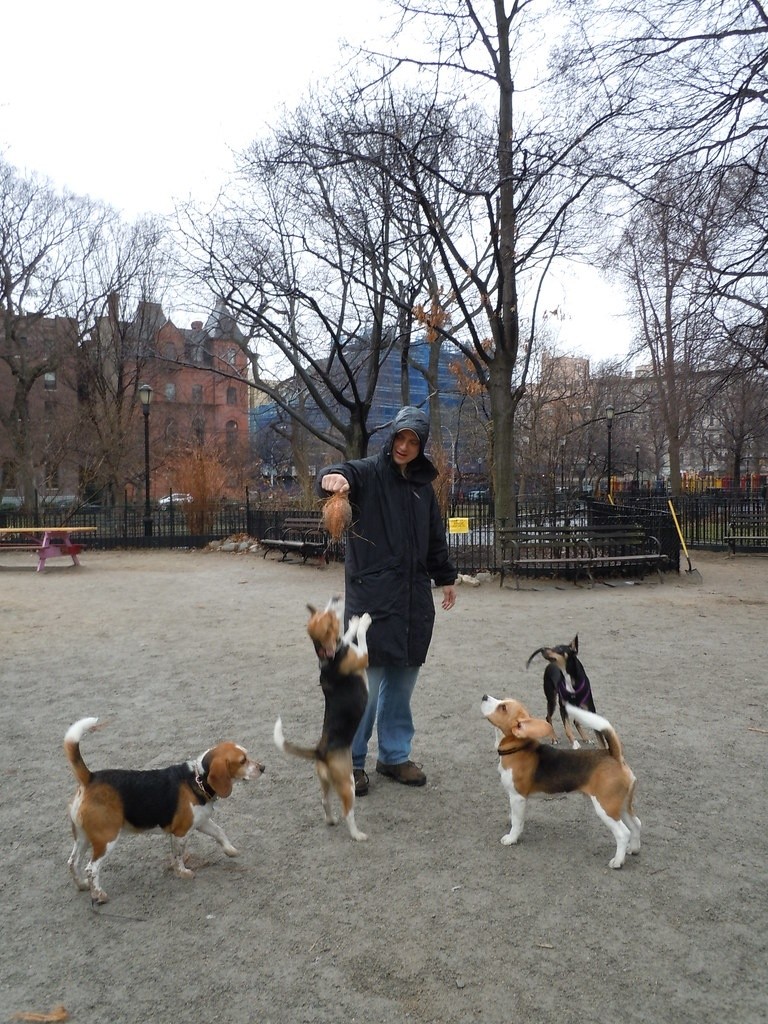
[353,769,369,796]
[376,760,427,786]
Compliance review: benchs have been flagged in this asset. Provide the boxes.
[260,517,339,570]
[0,543,91,560]
[498,523,669,591]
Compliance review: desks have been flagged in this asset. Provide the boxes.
[0,526,97,572]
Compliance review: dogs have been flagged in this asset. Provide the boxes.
[478,632,643,872]
[62,716,266,910]
[272,592,373,841]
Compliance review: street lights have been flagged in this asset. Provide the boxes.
[605,404,614,498]
[139,385,154,547]
[635,445,641,492]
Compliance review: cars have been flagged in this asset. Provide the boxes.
[158,493,194,511]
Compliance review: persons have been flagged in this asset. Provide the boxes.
[316,407,458,796]
